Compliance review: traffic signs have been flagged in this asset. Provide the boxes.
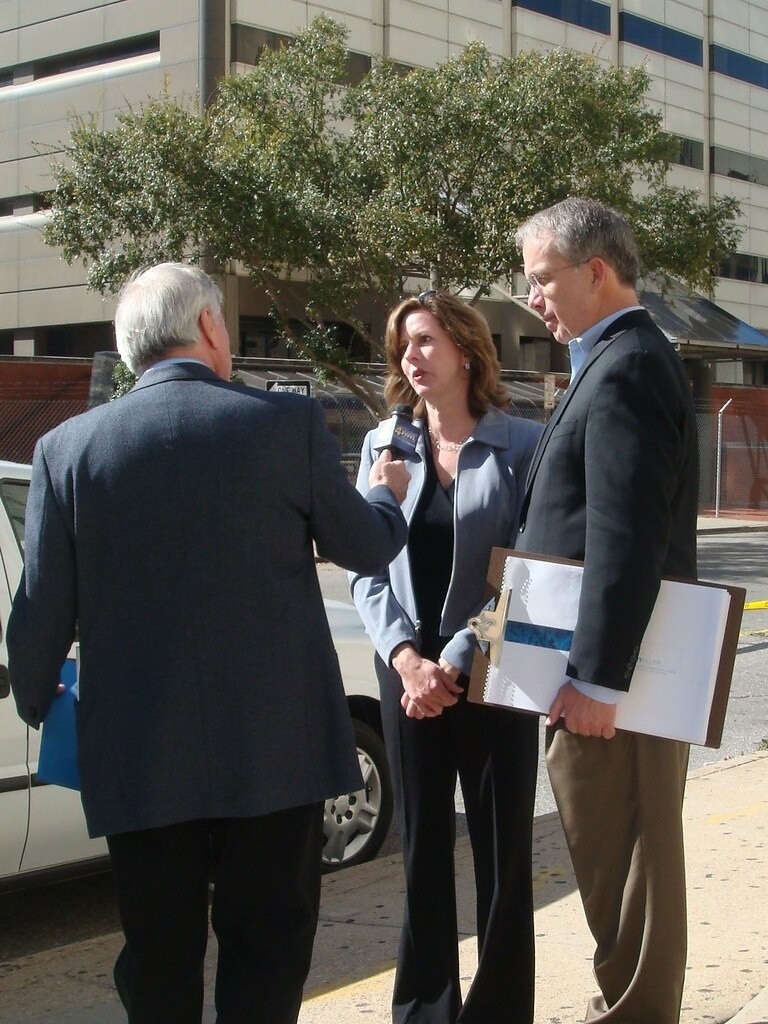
[265,379,311,398]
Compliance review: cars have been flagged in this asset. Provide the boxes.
[0,455,395,895]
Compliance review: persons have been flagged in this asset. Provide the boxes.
[6,263,410,1024]
[514,198,697,1024]
[348,294,547,1024]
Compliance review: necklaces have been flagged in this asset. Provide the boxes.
[428,422,475,451]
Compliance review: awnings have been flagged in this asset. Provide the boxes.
[496,263,768,359]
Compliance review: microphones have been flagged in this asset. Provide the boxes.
[373,403,421,463]
